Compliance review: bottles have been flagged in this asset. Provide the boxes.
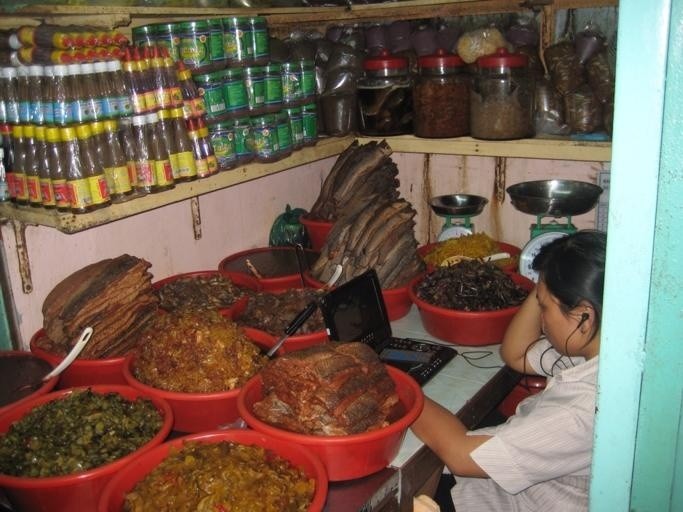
[0,22,218,215]
[357,45,536,142]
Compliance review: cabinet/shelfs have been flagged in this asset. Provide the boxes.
[0,0,619,292]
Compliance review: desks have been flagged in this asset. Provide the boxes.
[167,303,523,511]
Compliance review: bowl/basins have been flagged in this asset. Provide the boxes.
[29,310,167,387]
[123,325,283,434]
[99,430,329,512]
[4,384,171,510]
[304,260,420,323]
[239,353,425,483]
[495,375,546,420]
[152,267,246,321]
[217,244,317,291]
[239,290,332,352]
[410,235,541,347]
[0,349,61,416]
[295,212,336,245]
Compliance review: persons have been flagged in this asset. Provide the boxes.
[409,228,606,512]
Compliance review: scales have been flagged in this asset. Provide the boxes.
[506,179,603,286]
[428,194,488,242]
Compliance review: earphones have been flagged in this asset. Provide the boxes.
[577,313,589,328]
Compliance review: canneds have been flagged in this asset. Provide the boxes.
[132,16,318,171]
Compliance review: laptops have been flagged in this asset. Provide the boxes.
[317,268,458,388]
